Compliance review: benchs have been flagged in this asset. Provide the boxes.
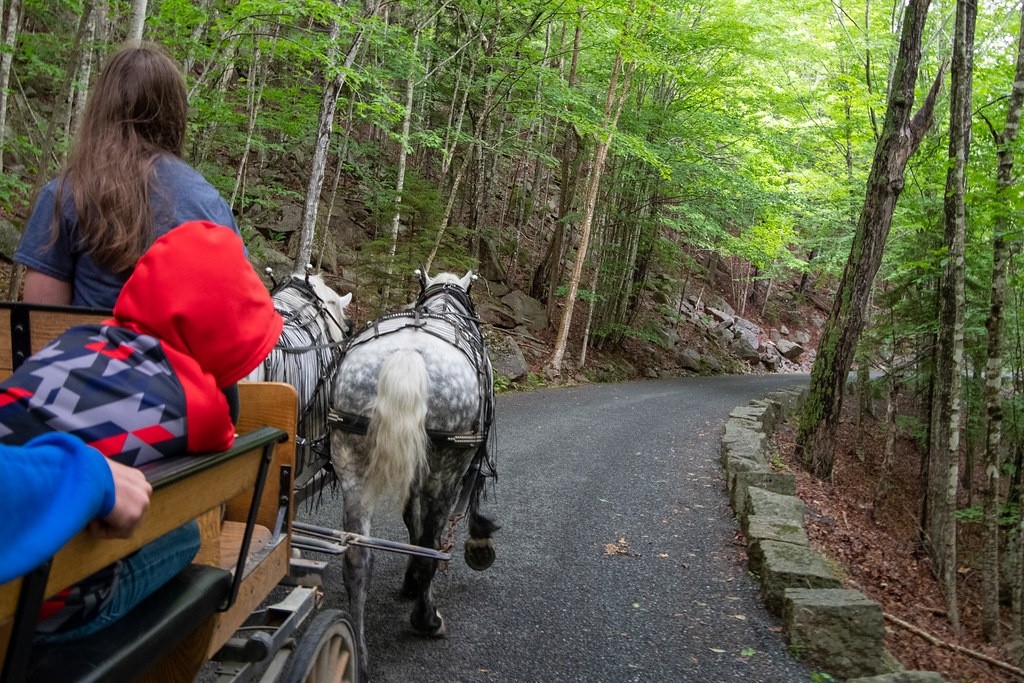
[0,301,300,683]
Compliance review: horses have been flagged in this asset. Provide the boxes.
[235,272,352,559]
[323,259,504,682]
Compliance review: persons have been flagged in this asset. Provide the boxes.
[0,432,154,583]
[0,219,284,475]
[8,48,246,309]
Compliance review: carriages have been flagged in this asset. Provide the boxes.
[1,253,504,682]
